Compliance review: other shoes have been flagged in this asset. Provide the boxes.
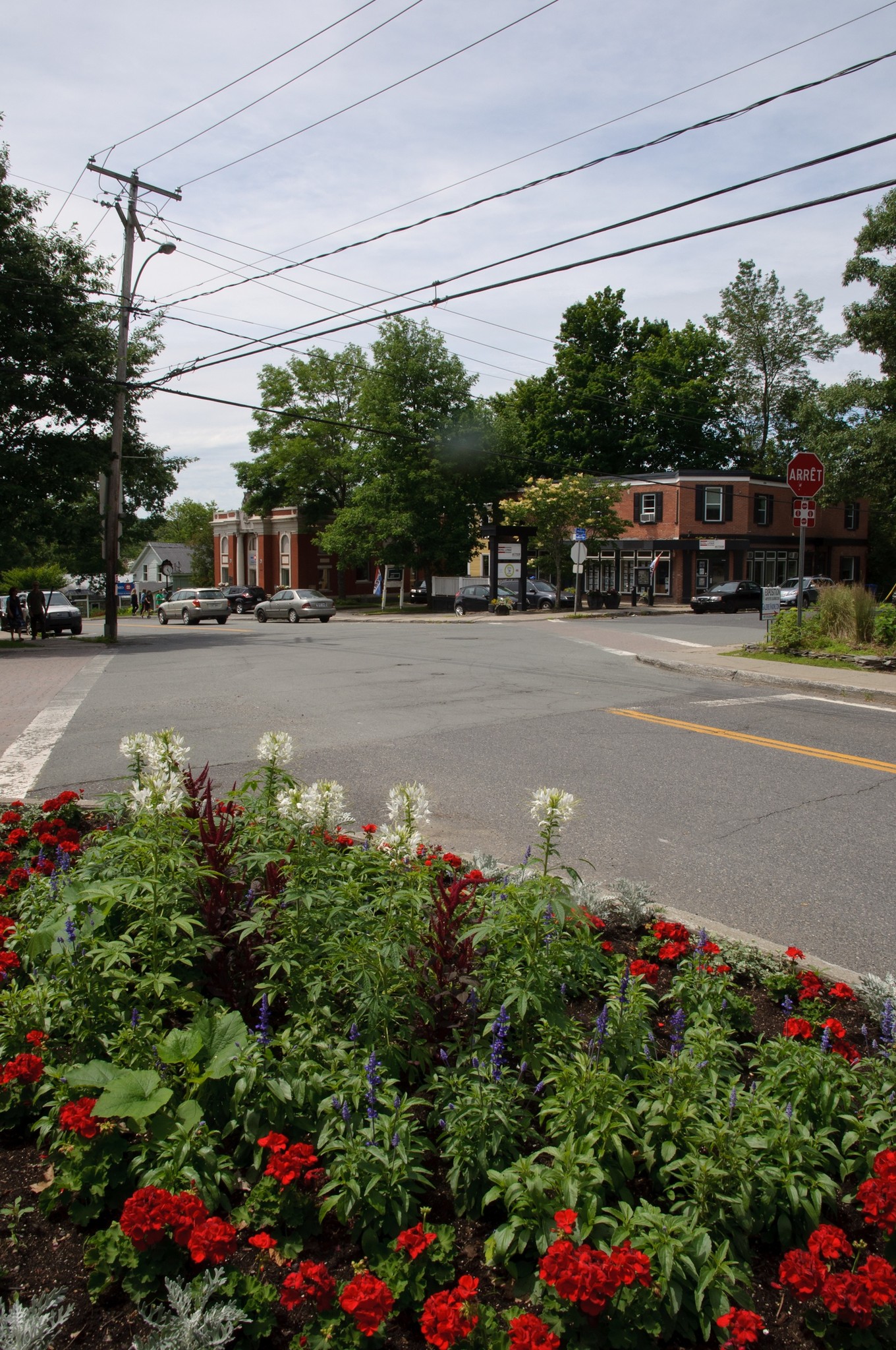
[42,633,49,639]
[141,615,143,618]
[133,613,136,616]
[19,638,24,641]
[31,637,36,640]
[147,616,151,618]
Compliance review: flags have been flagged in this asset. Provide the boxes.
[373,564,382,595]
[651,551,663,578]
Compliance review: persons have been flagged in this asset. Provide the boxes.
[140,588,151,618]
[156,588,181,609]
[131,588,139,616]
[6,586,24,641]
[26,581,48,640]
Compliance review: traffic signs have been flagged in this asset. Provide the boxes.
[760,587,781,621]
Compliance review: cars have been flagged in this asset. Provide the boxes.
[410,580,428,603]
[254,588,336,623]
[777,574,837,609]
[690,580,761,614]
[453,584,533,617]
[0,595,11,632]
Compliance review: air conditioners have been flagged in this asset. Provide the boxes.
[641,513,655,522]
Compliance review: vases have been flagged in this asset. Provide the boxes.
[640,597,648,604]
[603,595,621,609]
[494,606,510,615]
[587,595,604,609]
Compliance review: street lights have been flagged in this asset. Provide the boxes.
[104,241,178,643]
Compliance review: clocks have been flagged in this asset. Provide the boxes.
[163,565,173,575]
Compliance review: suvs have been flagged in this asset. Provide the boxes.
[217,584,268,614]
[158,587,232,625]
[16,588,82,636]
[500,578,576,609]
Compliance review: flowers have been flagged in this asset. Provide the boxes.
[586,588,657,596]
[492,596,515,610]
